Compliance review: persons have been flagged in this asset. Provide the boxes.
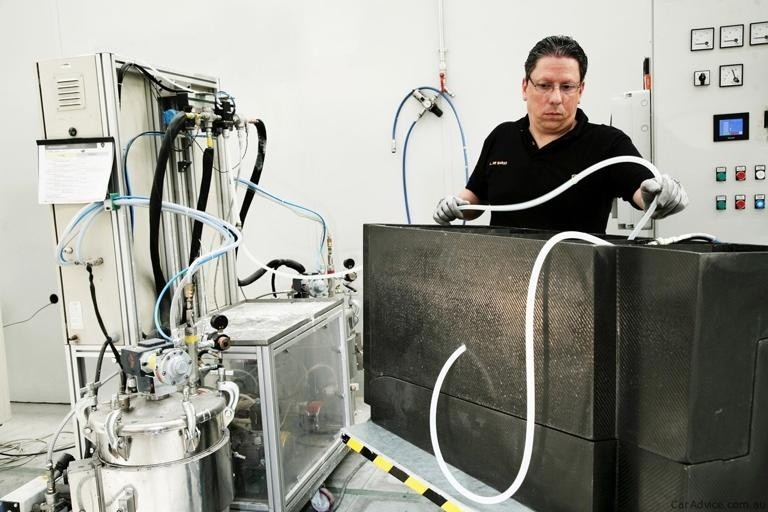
[430,33,688,234]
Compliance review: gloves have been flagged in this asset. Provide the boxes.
[432,195,470,225]
[638,174,690,219]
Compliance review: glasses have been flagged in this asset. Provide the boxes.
[525,74,584,94]
